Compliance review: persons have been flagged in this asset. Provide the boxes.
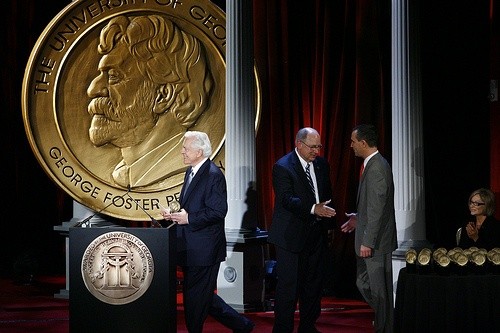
[160,130,258,333]
[460,188,500,250]
[400,327,401,329]
[86,15,215,188]
[265,126,337,333]
[340,124,400,333]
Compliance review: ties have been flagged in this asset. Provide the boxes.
[360,162,364,177]
[183,171,194,201]
[305,164,316,201]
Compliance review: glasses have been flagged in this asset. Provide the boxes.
[301,140,323,150]
[468,201,485,206]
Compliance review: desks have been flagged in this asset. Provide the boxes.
[395,269,500,332]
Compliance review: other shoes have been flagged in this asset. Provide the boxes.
[233,315,253,333]
